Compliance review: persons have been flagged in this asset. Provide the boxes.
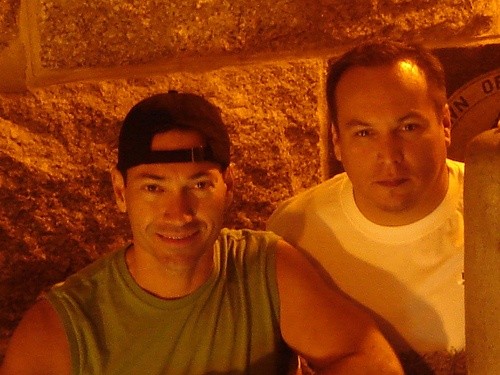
[263,40,466,375]
[0,87,406,375]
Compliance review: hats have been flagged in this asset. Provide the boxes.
[116,89,232,169]
